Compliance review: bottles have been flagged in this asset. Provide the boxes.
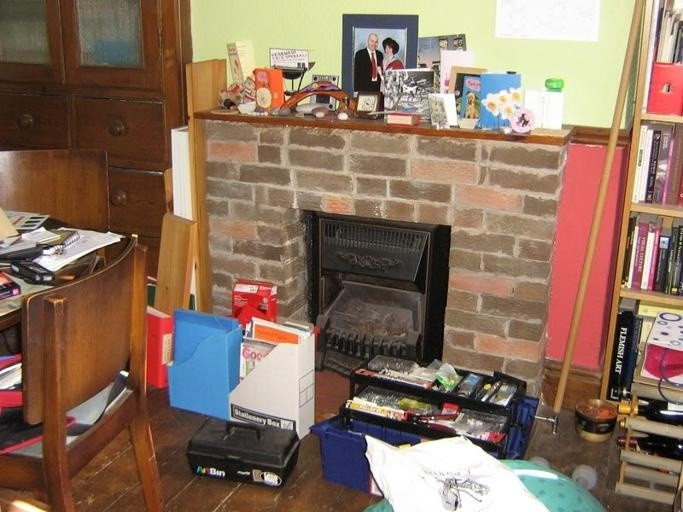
[617,386,683,476]
[542,78,563,130]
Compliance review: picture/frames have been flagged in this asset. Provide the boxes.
[341,14,419,104]
[447,65,487,122]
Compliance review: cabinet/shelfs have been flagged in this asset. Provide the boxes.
[0,0,193,284]
[600,0,683,423]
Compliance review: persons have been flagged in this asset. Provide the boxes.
[375,37,405,93]
[352,33,382,92]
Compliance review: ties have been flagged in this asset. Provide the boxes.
[371,53,377,79]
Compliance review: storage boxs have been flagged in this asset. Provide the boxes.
[310,364,540,499]
[187,417,300,488]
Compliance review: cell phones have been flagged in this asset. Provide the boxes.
[11,259,55,283]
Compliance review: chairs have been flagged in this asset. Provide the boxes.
[0,231,167,512]
[0,148,110,270]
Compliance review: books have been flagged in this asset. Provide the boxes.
[0,204,126,317]
[237,312,315,386]
[0,351,23,391]
[380,30,524,130]
[143,302,174,390]
[605,0,681,428]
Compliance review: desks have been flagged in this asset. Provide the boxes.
[0,209,98,357]
